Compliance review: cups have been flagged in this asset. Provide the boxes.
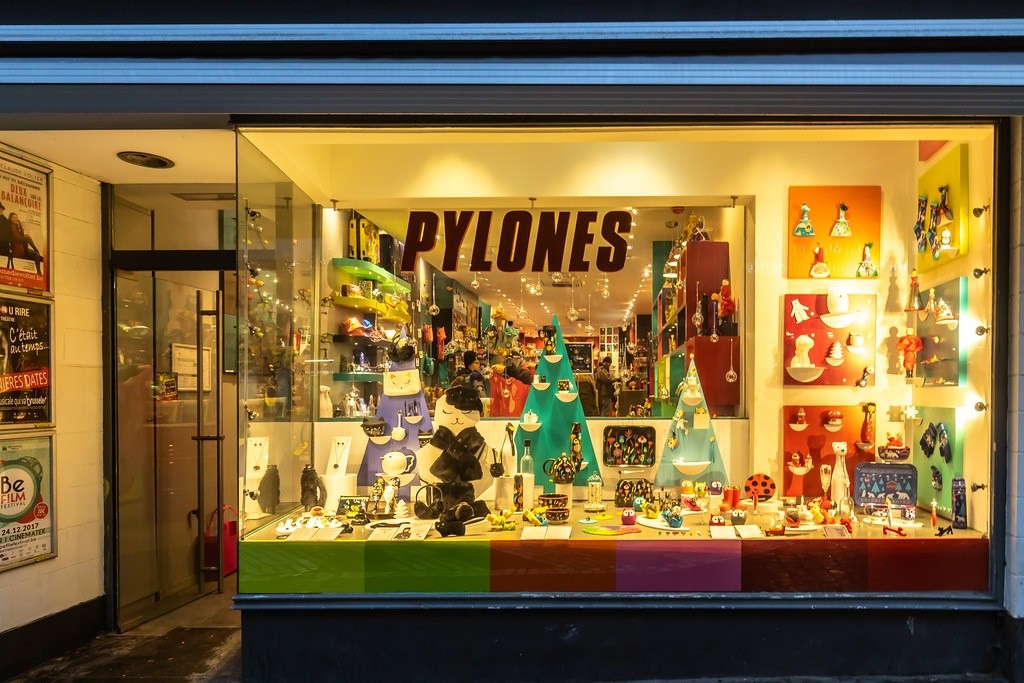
[901,506,916,523]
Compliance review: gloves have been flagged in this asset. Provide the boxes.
[919,423,937,458]
[937,423,951,463]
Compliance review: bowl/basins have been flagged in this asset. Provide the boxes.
[878,446,910,461]
[538,494,568,508]
[546,508,570,525]
[866,505,888,517]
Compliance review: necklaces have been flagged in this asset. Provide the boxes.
[334,438,349,468]
[250,438,267,470]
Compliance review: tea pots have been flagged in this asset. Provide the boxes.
[542,453,584,484]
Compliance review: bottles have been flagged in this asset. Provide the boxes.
[501,423,518,476]
[952,478,967,529]
[839,475,854,517]
[587,470,603,505]
[520,439,533,474]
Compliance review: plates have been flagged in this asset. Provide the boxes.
[365,519,414,530]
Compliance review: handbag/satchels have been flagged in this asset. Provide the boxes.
[383,370,420,397]
[199,504,238,580]
[420,324,434,343]
[422,358,435,376]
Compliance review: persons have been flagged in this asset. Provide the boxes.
[455,351,531,398]
[594,356,617,417]
[118,362,152,434]
[897,327,923,378]
[0,200,43,274]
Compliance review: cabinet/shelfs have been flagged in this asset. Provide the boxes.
[328,241,738,418]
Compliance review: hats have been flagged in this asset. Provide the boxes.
[603,357,612,363]
[464,351,477,368]
[507,347,525,359]
